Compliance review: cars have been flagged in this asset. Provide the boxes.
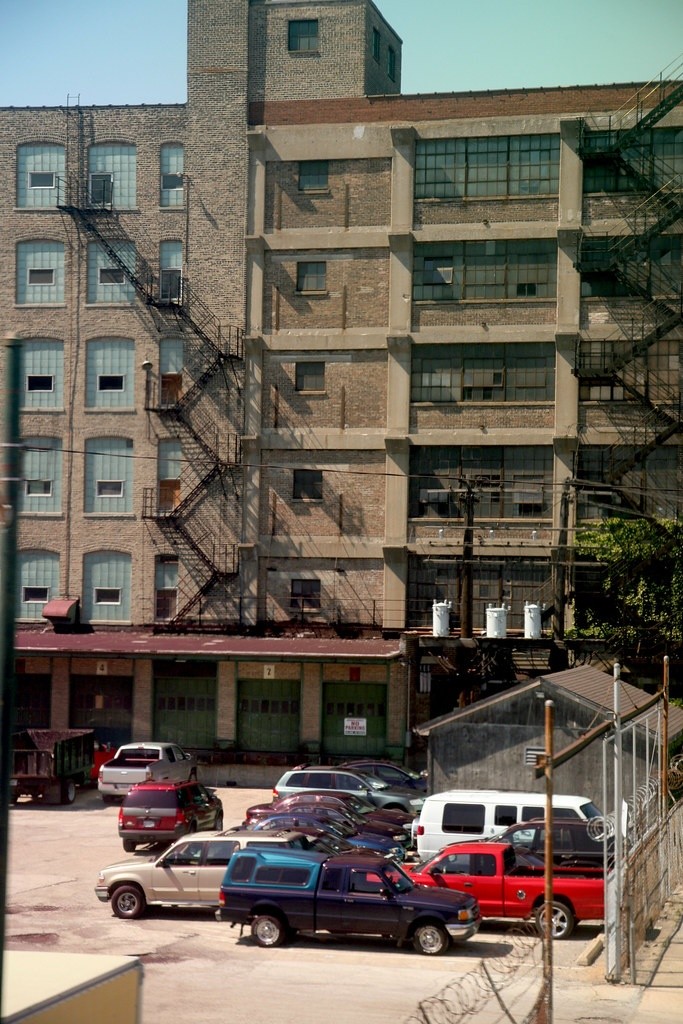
[231,792,417,861]
[337,760,428,797]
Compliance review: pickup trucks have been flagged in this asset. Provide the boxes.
[95,741,198,807]
[213,854,483,955]
[364,843,615,939]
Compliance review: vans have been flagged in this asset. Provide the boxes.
[407,788,611,867]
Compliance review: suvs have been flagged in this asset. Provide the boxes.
[271,760,428,817]
[94,831,310,918]
[117,778,226,854]
[442,818,631,882]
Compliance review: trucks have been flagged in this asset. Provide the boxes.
[10,727,111,804]
